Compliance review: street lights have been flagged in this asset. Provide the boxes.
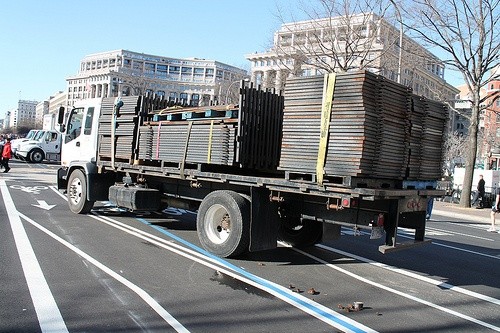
[225,78,250,105]
[111,81,137,96]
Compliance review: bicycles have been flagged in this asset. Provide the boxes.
[434,180,480,209]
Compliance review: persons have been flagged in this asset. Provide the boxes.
[486,179,500,233]
[0,131,12,173]
[471,175,485,208]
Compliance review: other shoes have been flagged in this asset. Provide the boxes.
[487,227,496,232]
[3,167,10,173]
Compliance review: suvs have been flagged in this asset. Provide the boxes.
[10,130,61,163]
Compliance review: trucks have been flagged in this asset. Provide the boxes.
[57,98,446,257]
